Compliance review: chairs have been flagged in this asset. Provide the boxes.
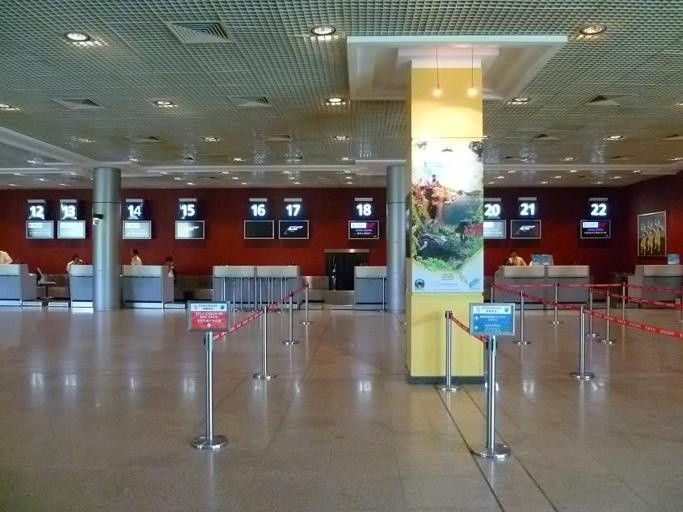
[37,268,57,299]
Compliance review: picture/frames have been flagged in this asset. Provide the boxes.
[636,211,667,257]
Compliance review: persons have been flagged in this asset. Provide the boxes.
[0,250,12,264]
[527,251,541,267]
[506,249,525,266]
[65,254,84,307]
[428,175,440,188]
[129,248,143,266]
[163,256,177,278]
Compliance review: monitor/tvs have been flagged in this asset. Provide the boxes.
[244,220,275,239]
[510,220,542,240]
[25,220,55,240]
[56,219,88,240]
[121,219,152,240]
[483,219,506,240]
[579,219,612,241]
[667,253,680,265]
[532,255,554,266]
[278,220,309,239]
[348,220,379,240]
[174,219,205,240]
